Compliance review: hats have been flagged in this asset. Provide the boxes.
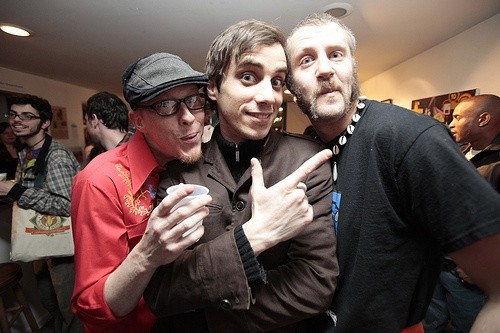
[121,52,210,106]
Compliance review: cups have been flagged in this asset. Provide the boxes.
[166,183,209,242]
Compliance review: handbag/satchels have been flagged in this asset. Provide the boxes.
[10,201,75,261]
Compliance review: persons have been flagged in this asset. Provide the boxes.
[142,19,338,332]
[0,92,137,333]
[423,93,500,333]
[284,13,500,333]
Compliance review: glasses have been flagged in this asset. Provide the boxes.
[4,112,41,120]
[135,93,207,116]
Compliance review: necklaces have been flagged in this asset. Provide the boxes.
[333,94,367,181]
